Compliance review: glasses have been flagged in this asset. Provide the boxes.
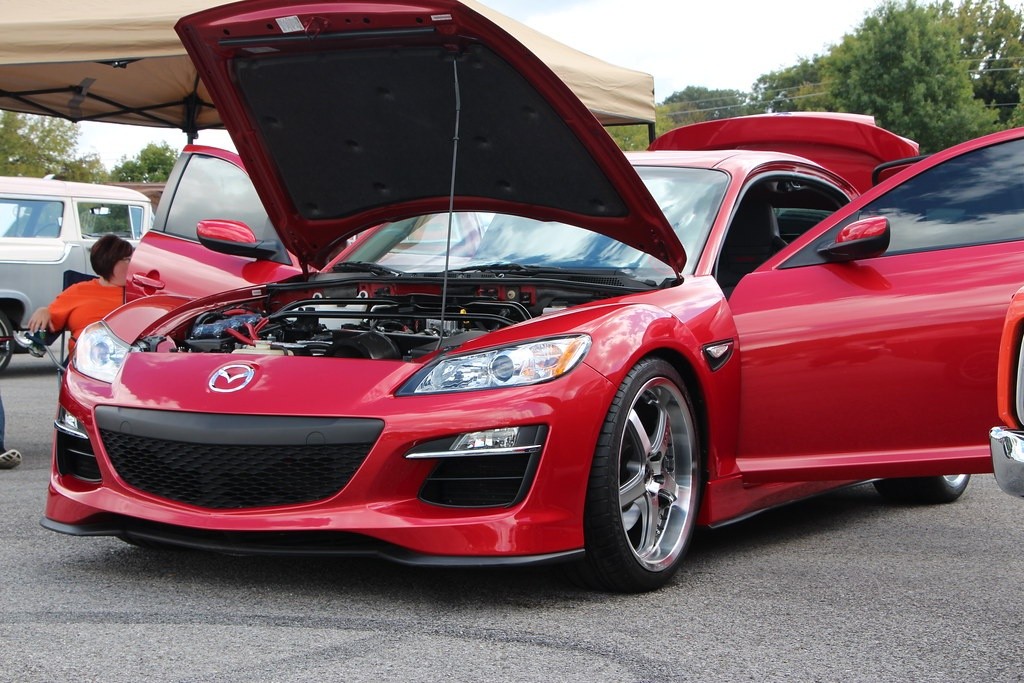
[120,257,131,263]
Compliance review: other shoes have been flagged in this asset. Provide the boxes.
[0,448,22,470]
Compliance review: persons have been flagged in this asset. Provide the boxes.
[0,395,22,468]
[27,233,135,358]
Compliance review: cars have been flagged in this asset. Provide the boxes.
[36,0,1024,596]
[1,176,156,375]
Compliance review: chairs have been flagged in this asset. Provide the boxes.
[701,184,788,287]
[28,269,100,396]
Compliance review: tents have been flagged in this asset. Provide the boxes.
[0,0,657,145]
